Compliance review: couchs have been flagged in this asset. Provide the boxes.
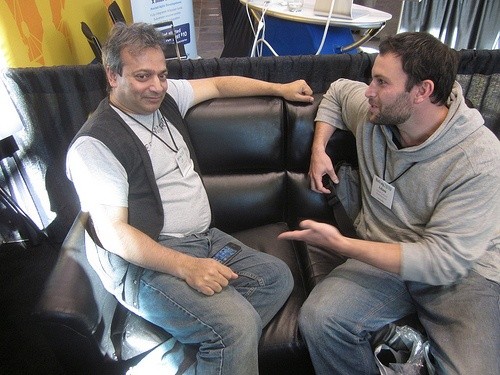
[39,94,500,375]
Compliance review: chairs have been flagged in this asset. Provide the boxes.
[107,1,187,60]
[81,22,103,64]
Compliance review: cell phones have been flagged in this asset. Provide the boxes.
[210,242,241,265]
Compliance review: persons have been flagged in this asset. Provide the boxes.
[63,20,315,375]
[277,31,500,375]
